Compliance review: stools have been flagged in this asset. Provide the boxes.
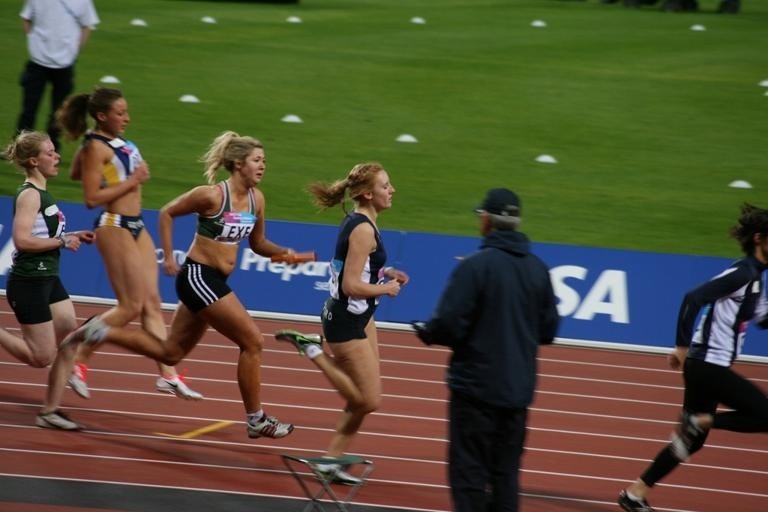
[280,453,375,512]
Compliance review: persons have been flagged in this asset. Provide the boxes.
[52,85,210,403]
[614,197,767,512]
[14,0,103,155]
[411,185,561,512]
[0,129,86,433]
[57,127,298,440]
[270,159,412,486]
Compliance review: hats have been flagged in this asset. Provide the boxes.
[473,187,521,217]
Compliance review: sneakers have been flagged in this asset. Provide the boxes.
[617,488,655,511]
[245,416,294,438]
[274,328,323,353]
[673,409,706,459]
[67,362,90,399]
[33,410,81,430]
[59,314,107,348]
[312,465,361,486]
[156,375,204,401]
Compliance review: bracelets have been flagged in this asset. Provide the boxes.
[382,265,392,275]
[54,233,68,250]
